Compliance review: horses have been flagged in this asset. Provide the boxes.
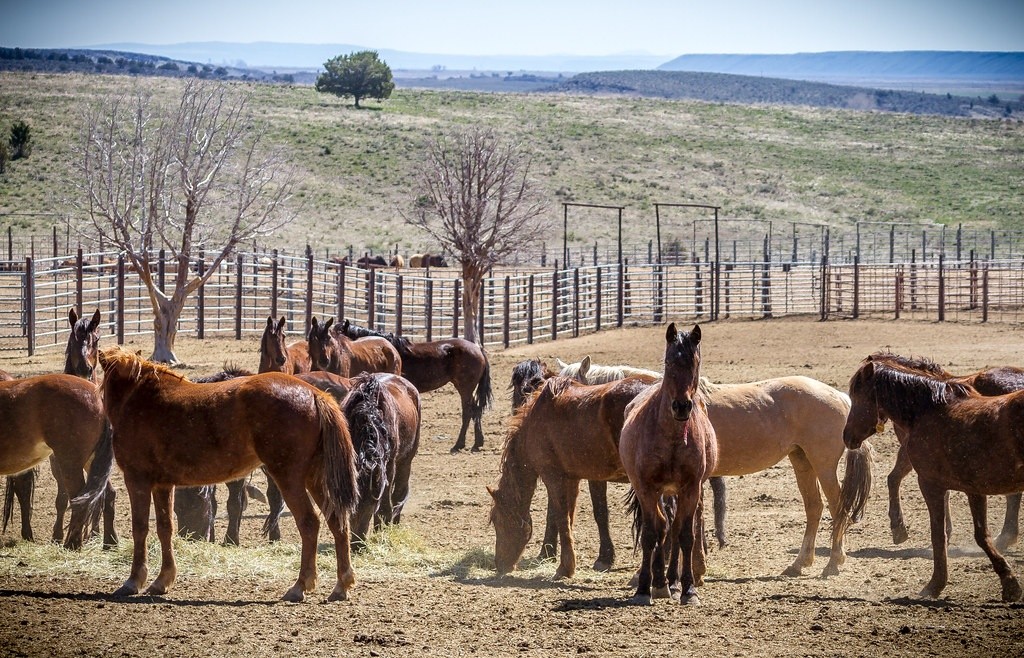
[0,308,493,602]
[842,351,1023,605]
[483,318,872,604]
[0,246,454,281]
